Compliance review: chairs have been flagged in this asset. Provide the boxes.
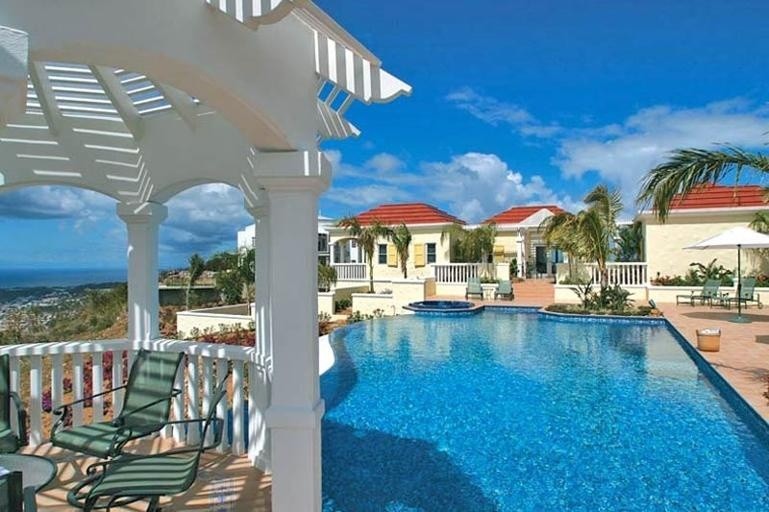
[464,276,514,300]
[0,346,232,511]
[676,277,764,310]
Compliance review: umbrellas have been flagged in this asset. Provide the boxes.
[681,226,769,316]
